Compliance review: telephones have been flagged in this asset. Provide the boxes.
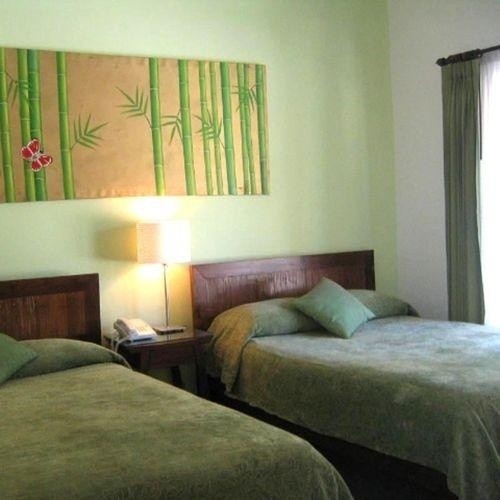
[114,318,157,342]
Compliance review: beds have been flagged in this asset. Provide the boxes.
[190,249,495,499]
[0,273,353,500]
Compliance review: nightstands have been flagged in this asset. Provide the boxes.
[103,323,213,403]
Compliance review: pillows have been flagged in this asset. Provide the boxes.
[278,276,376,339]
[0,333,41,385]
